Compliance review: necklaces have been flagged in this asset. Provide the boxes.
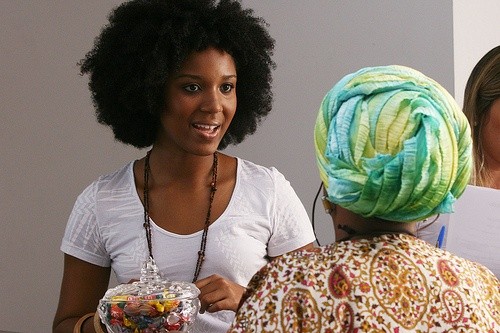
[143,147,219,283]
[334,221,417,242]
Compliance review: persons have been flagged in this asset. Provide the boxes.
[223,64,500,332]
[462,45,500,191]
[52,0,316,333]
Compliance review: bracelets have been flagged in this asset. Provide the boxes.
[93,309,104,332]
[72,310,95,332]
